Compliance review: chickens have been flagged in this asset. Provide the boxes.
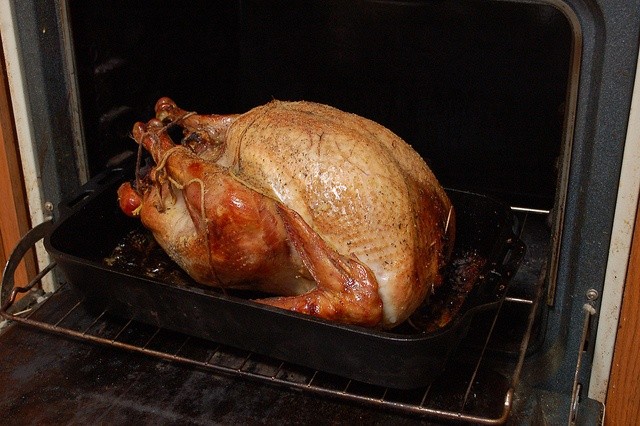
[115,96,458,330]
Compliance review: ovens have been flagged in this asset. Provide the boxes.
[1,1,640,424]
